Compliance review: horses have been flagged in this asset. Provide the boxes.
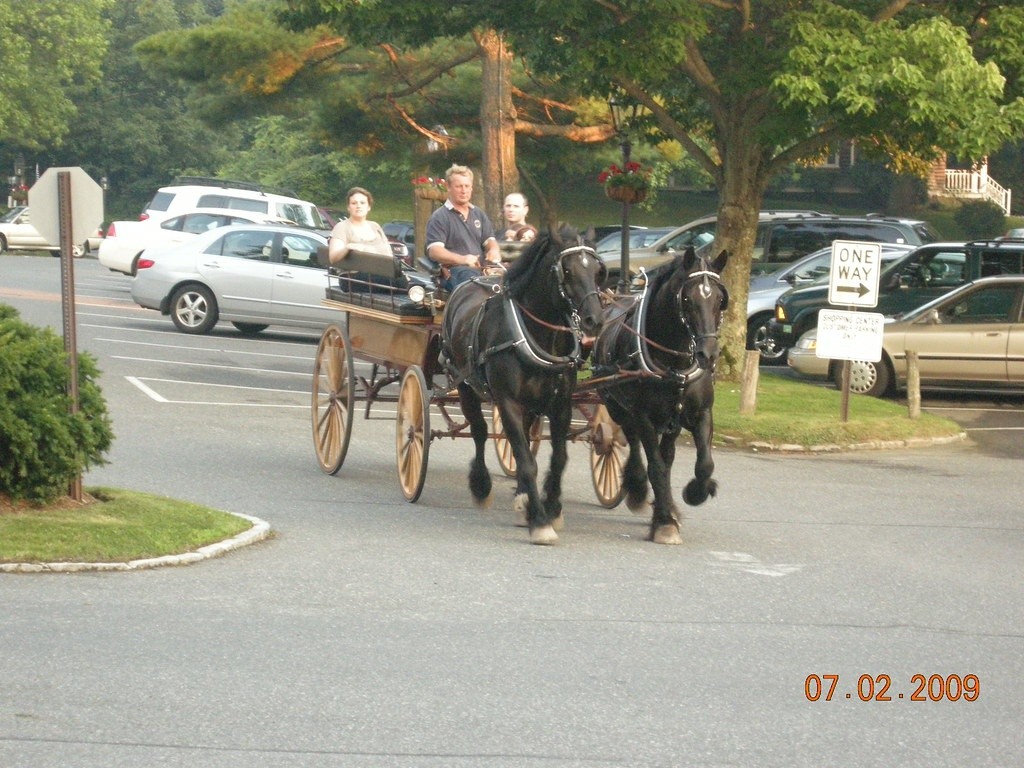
[590,243,731,548]
[440,207,607,541]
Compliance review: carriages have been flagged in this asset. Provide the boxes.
[309,220,730,548]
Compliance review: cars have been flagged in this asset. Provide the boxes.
[590,208,1024,399]
[0,204,89,259]
[95,184,416,341]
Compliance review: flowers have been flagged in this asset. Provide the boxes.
[599,163,658,211]
[412,176,447,188]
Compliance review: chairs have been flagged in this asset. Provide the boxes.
[282,247,290,263]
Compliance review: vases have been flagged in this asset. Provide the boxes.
[606,185,646,204]
[415,188,447,200]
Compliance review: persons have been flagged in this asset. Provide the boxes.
[495,191,539,242]
[424,163,502,293]
[328,187,426,295]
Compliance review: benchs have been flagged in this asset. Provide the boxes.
[317,243,530,327]
